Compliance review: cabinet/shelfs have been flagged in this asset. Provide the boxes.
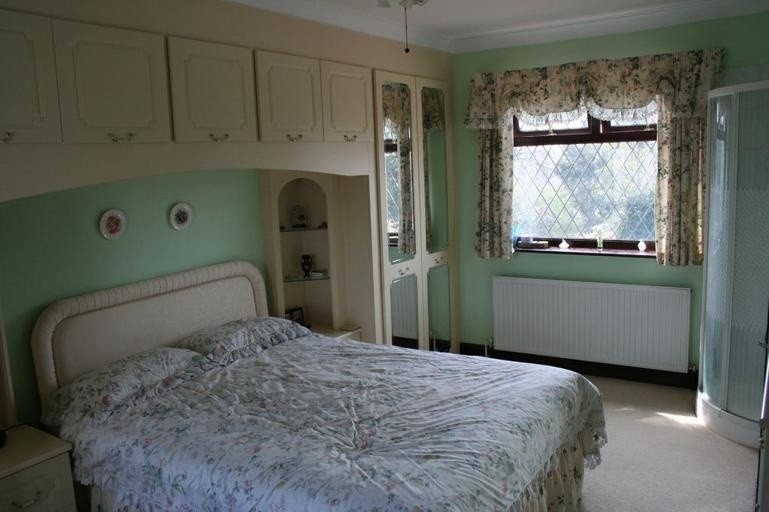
[0,0,62,203]
[53,0,257,191]
[371,35,461,353]
[253,8,373,177]
[260,170,348,330]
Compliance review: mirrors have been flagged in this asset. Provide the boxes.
[381,80,451,353]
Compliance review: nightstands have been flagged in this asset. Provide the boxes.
[0,425,77,512]
[311,326,362,341]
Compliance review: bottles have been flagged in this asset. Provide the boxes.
[596,229,603,248]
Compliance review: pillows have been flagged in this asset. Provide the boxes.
[178,316,312,366]
[49,347,219,425]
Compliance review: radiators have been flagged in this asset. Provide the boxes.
[490,276,691,374]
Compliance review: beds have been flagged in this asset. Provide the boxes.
[33,260,608,512]
